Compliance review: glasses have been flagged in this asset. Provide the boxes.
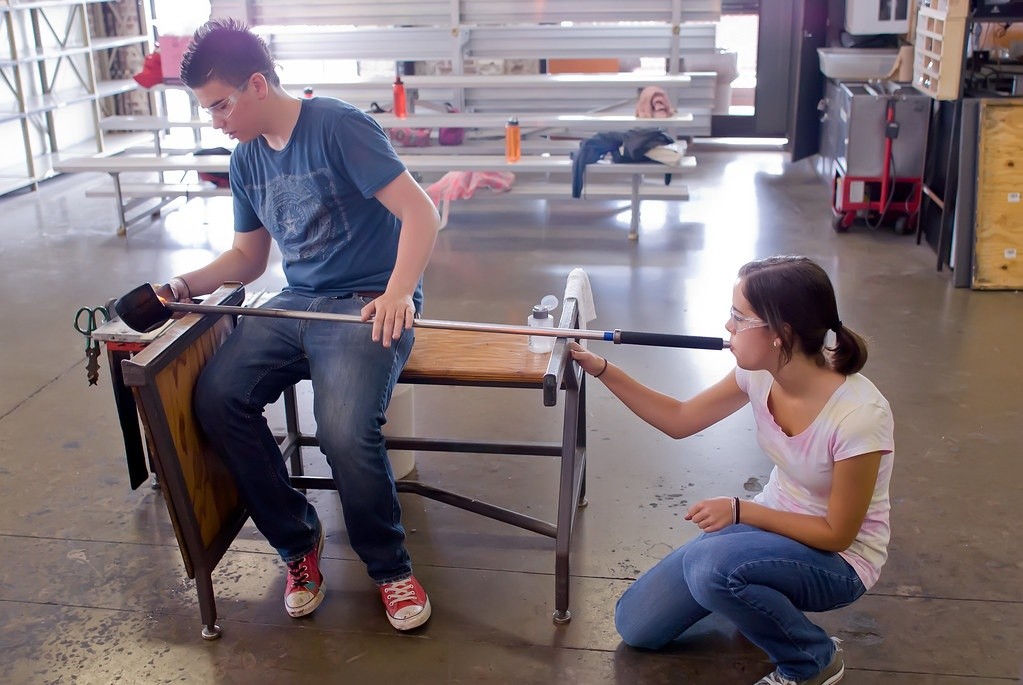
[199,69,269,119]
[730,306,769,332]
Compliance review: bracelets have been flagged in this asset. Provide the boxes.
[594,359,607,378]
[731,497,740,525]
[175,276,190,298]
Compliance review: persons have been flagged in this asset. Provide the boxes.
[571,254,895,685]
[150,20,439,630]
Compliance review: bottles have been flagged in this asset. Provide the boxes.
[528,305,554,353]
[304,88,312,98]
[392,77,405,117]
[505,116,521,164]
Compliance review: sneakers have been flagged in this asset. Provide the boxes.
[283,520,326,617]
[379,573,432,631]
[752,636,845,685]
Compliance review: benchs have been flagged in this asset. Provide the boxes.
[53,0,722,237]
[91,282,589,624]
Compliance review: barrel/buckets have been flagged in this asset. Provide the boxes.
[380,384,415,481]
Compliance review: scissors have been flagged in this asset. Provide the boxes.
[74,306,110,386]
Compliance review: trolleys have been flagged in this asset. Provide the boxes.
[832,98,921,238]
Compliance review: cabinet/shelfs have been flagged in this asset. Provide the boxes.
[0,0,170,198]
